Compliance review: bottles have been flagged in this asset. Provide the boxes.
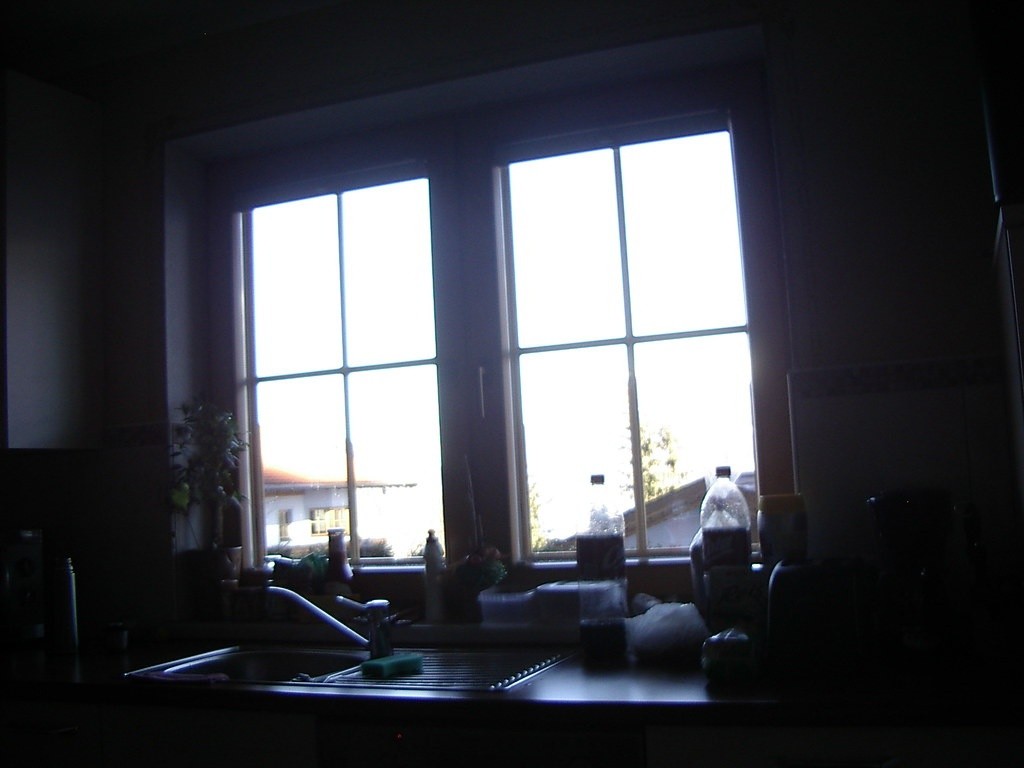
[575,475,628,579]
[700,466,754,597]
[426,532,441,579]
[689,503,743,602]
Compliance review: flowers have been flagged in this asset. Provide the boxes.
[165,385,242,546]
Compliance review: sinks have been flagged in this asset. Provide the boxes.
[159,649,365,686]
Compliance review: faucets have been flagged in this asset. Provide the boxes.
[257,582,402,657]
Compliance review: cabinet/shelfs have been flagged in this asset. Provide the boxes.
[0,645,1024,768]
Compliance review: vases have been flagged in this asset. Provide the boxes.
[185,545,244,584]
